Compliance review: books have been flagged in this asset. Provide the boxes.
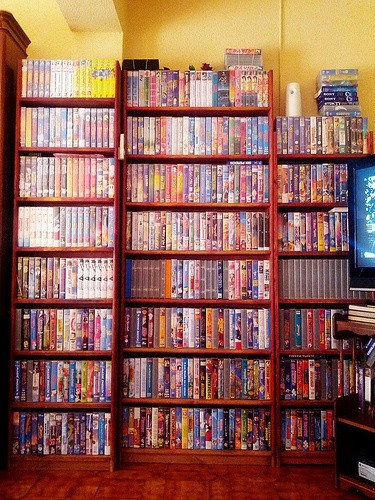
[13,49,375,455]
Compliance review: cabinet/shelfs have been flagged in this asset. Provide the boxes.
[0,8,375,500]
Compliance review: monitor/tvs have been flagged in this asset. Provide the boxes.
[347,153,375,291]
